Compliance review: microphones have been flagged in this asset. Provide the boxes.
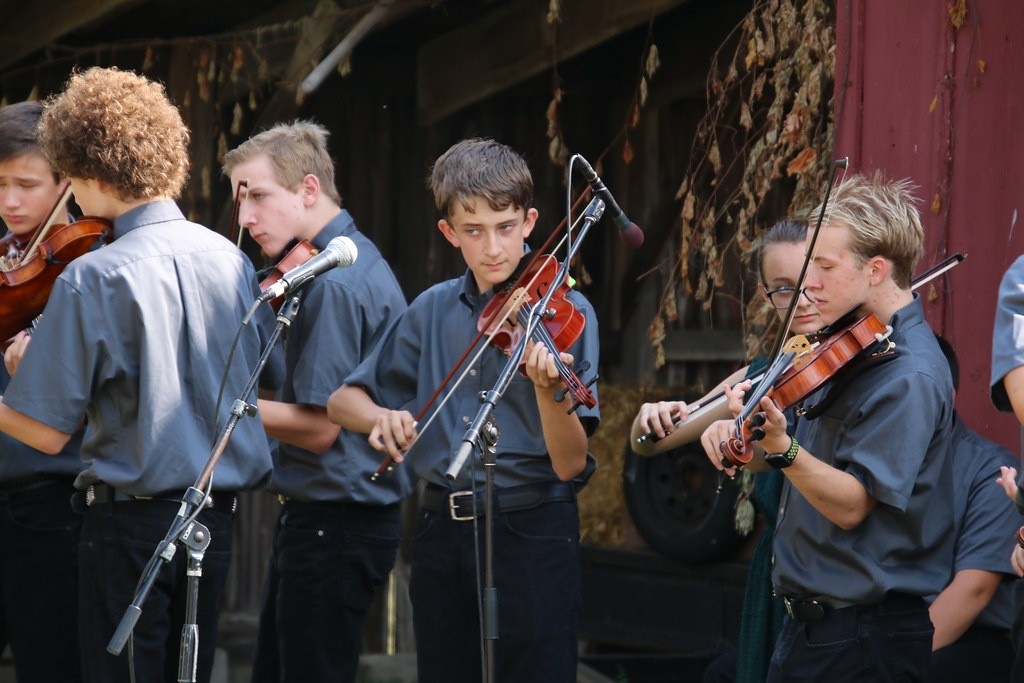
[575,153,644,248]
[258,236,358,303]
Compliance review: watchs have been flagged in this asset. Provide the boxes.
[763,436,799,470]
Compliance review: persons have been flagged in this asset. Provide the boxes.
[990,252,1024,581]
[328,138,603,683]
[0,65,285,683]
[628,171,1024,681]
[221,118,408,683]
[0,99,114,406]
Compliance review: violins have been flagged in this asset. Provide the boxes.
[1,216,114,345]
[718,313,889,471]
[473,250,602,416]
[259,235,316,305]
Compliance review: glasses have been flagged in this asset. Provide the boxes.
[763,284,819,309]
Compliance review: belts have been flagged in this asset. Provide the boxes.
[1,474,63,503]
[783,591,913,622]
[85,481,237,516]
[420,483,579,521]
